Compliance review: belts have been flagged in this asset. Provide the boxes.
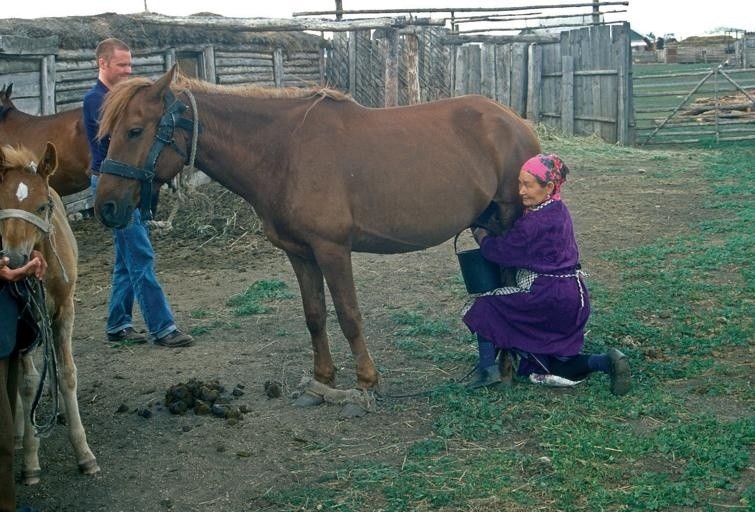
[92,171,100,176]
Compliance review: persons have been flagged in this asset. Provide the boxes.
[82,34,195,351]
[0,246,49,286]
[461,151,630,399]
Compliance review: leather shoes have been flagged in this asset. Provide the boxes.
[107,326,148,343]
[153,329,195,347]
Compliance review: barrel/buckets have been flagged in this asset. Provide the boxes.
[454,222,502,294]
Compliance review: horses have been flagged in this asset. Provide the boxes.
[0,142,100,486]
[94,61,542,421]
[0,83,159,220]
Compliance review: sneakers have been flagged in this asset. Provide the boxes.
[606,346,633,395]
[463,364,504,391]
